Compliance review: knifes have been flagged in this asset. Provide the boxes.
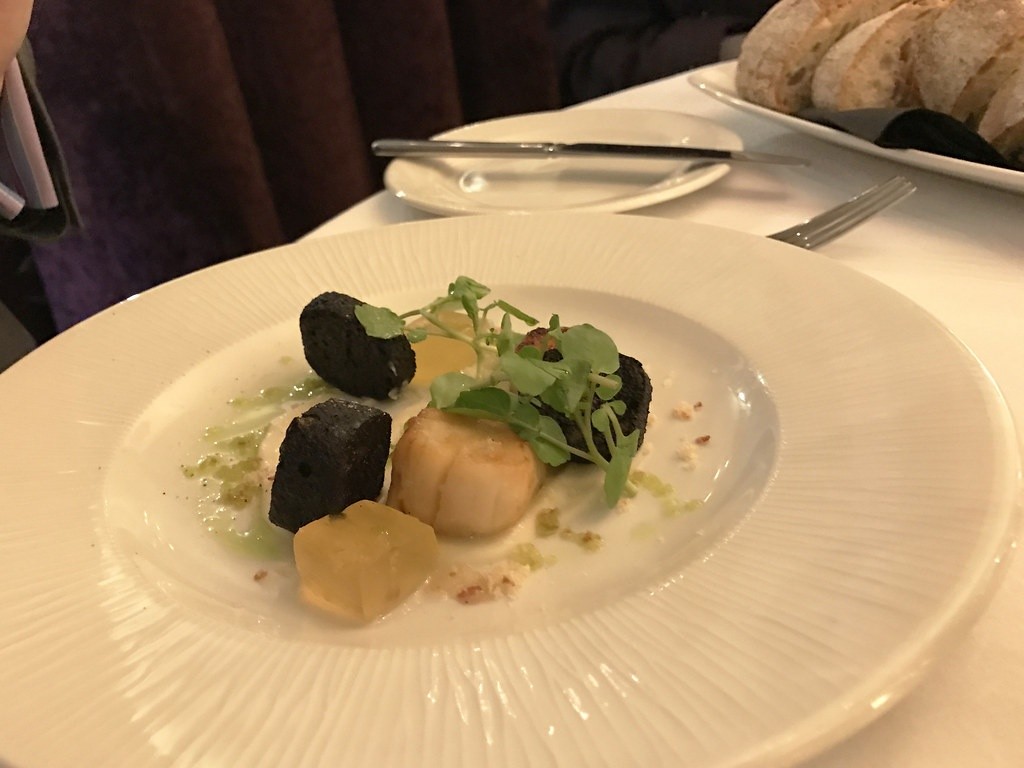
[371,136,809,166]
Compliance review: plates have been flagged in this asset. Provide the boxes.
[687,62,1024,196]
[384,109,744,215]
[2,209,1023,768]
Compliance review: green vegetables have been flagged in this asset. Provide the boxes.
[356,275,646,507]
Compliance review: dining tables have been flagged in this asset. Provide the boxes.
[294,64,1024,768]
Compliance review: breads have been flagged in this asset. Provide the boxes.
[736,0,1024,168]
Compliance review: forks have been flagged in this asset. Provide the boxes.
[767,171,918,249]
